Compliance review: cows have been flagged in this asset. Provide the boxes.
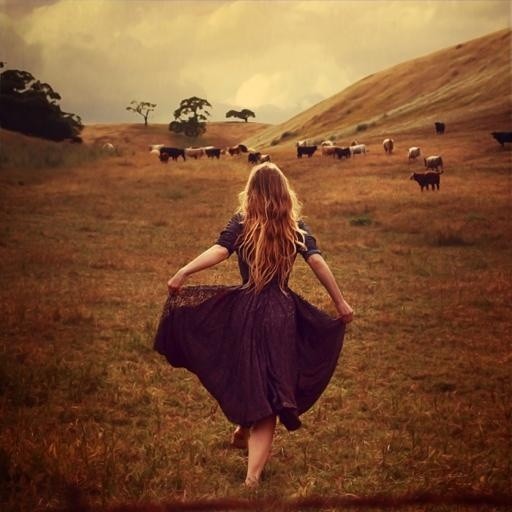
[407,147,421,161]
[434,121,445,135]
[409,170,444,192]
[248,151,271,165]
[383,138,394,154]
[296,139,317,158]
[148,144,256,163]
[424,155,443,171]
[490,131,512,147]
[321,138,366,160]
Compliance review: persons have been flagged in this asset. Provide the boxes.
[165,162,357,488]
